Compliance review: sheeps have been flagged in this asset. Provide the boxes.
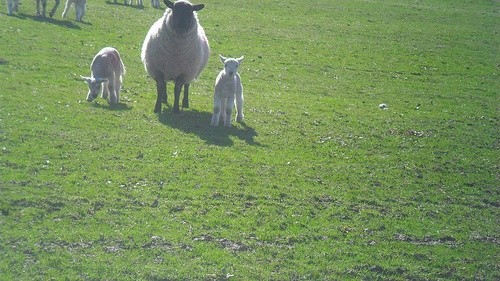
[79,47,126,104]
[210,54,245,128]
[140,0,210,113]
